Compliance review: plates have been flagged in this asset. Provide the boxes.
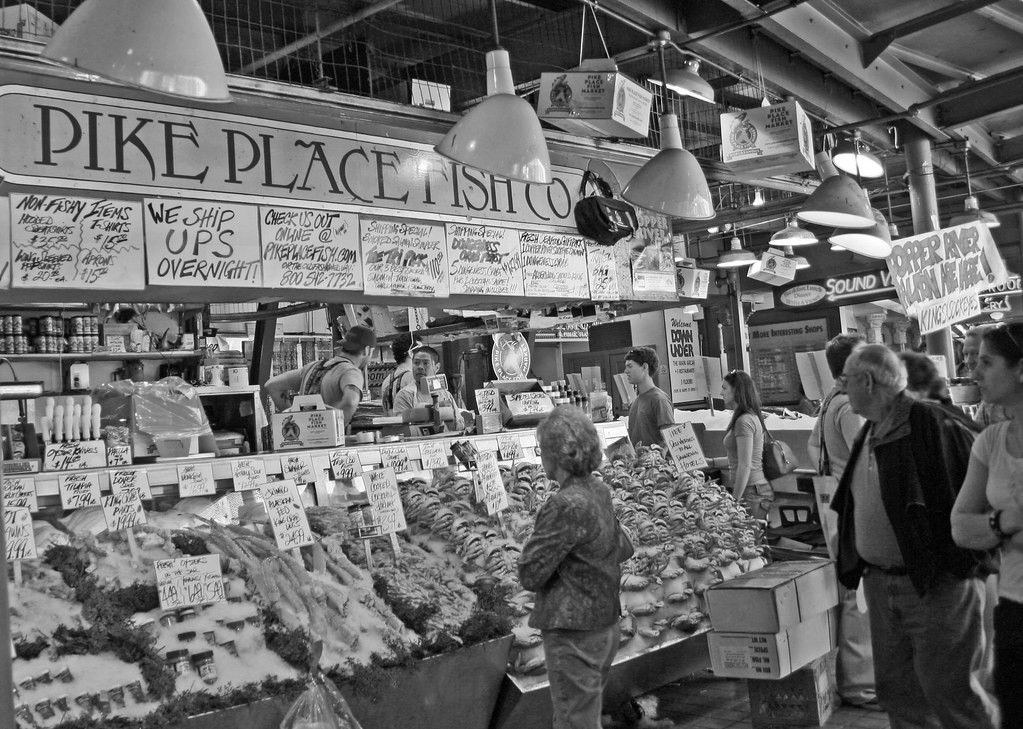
[491,332,530,380]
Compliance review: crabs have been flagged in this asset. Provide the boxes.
[394,441,775,678]
[181,512,412,675]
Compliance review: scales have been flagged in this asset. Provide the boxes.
[383,374,450,437]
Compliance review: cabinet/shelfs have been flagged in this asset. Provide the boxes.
[562,345,659,419]
[0,350,260,469]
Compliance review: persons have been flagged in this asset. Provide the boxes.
[949,321,1023,729]
[830,342,1001,729]
[806,332,979,729]
[624,346,675,460]
[703,369,775,564]
[264,325,377,435]
[381,332,465,435]
[517,404,622,729]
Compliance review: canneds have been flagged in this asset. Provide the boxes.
[0,314,99,354]
[541,384,589,415]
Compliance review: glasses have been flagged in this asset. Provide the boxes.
[837,372,862,386]
[730,368,738,378]
[995,320,1020,347]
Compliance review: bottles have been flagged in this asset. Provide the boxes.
[203,631,215,645]
[178,631,197,642]
[942,377,977,420]
[136,343,141,352]
[142,333,149,352]
[542,385,589,414]
[191,650,217,684]
[140,619,160,638]
[30,316,99,353]
[165,649,191,674]
[178,607,196,622]
[0,315,28,353]
[200,603,259,630]
[220,640,239,659]
[158,611,177,626]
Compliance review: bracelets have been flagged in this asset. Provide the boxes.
[988,510,1010,540]
[712,458,715,469]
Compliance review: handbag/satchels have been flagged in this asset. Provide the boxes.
[762,424,799,481]
[280,670,363,729]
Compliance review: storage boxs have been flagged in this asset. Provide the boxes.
[537,72,653,137]
[486,379,557,429]
[272,395,345,451]
[703,558,843,729]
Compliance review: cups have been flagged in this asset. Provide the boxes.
[28,345,34,352]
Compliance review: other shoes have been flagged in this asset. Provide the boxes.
[859,695,884,711]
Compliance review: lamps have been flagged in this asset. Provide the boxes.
[646,59,716,106]
[621,29,716,222]
[433,0,554,186]
[41,0,232,103]
[717,134,1001,269]
[683,305,698,313]
